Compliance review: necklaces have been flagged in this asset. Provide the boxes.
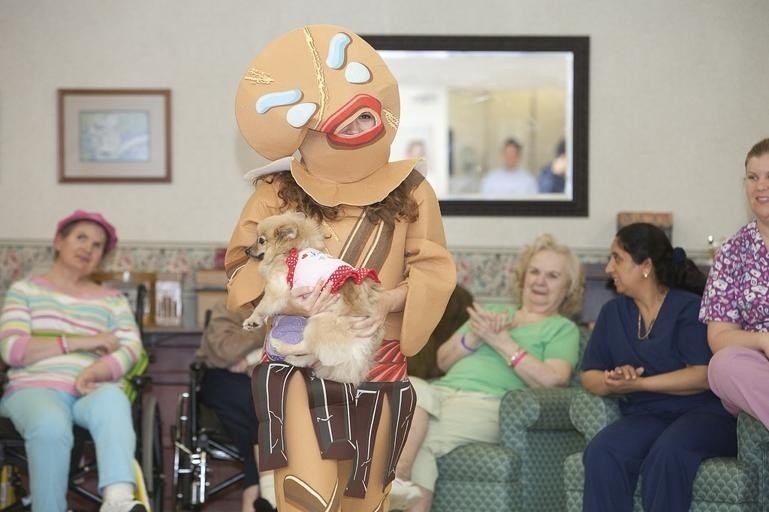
[637,286,670,341]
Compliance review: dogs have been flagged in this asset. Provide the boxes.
[242,208,390,390]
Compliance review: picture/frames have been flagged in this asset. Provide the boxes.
[55,87,173,187]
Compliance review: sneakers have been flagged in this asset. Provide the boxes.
[388,473,422,510]
[98,495,148,511]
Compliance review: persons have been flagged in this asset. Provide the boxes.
[197,297,267,511]
[698,139,768,426]
[387,236,585,510]
[578,222,737,512]
[223,25,459,511]
[1,209,149,512]
[406,136,566,196]
[389,285,475,501]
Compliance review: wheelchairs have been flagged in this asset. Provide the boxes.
[1,284,169,511]
[171,309,246,511]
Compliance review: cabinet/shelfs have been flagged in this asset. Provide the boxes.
[141,333,205,449]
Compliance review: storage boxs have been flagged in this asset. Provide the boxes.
[193,270,229,331]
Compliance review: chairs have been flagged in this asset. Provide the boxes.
[562,380,769,511]
[430,324,596,512]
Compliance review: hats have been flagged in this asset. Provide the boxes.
[53,208,119,255]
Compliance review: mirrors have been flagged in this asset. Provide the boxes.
[357,33,592,222]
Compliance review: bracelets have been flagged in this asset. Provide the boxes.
[460,336,476,353]
[56,334,65,354]
[62,335,69,354]
[510,351,526,369]
[508,348,521,366]
